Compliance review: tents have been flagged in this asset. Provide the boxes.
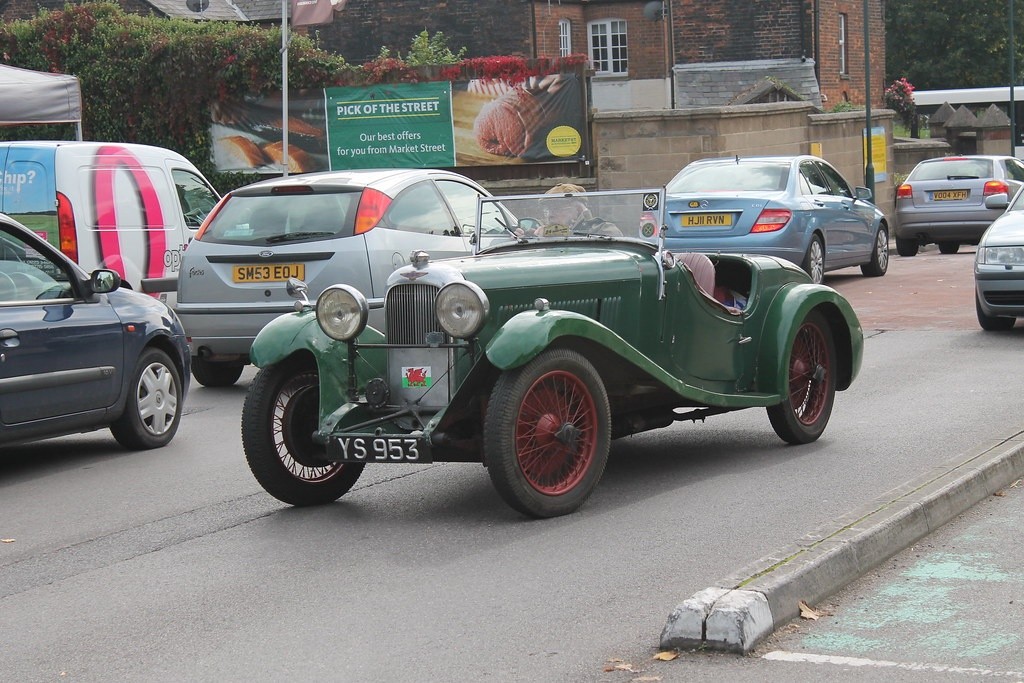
[0,64,83,141]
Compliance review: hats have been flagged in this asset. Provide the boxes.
[539,183,588,206]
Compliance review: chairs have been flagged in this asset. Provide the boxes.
[674,253,715,299]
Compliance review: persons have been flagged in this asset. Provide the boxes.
[509,184,623,242]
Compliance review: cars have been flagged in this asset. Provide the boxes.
[177,168,519,388]
[894,155,1024,257]
[974,183,1024,331]
[239,185,866,519]
[638,154,890,285]
[0,213,192,450]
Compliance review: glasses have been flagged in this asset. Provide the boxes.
[543,205,575,216]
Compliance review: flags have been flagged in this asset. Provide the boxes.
[292,0,333,28]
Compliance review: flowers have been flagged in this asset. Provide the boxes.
[884,78,915,112]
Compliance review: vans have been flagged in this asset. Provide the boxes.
[0,140,222,309]
